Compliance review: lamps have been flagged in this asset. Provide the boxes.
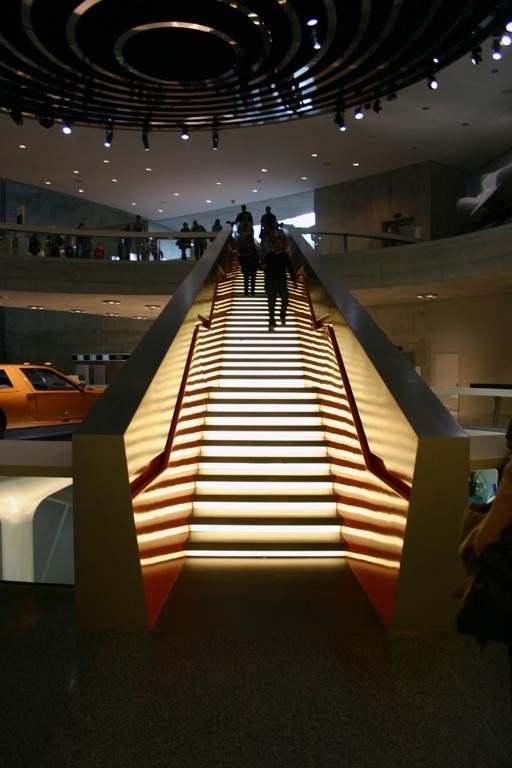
[9,103,219,151]
[309,28,512,131]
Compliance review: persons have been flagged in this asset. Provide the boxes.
[225,203,298,333]
[455,417,512,649]
[28,214,223,260]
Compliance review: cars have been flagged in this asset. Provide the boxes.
[1,357,111,437]
